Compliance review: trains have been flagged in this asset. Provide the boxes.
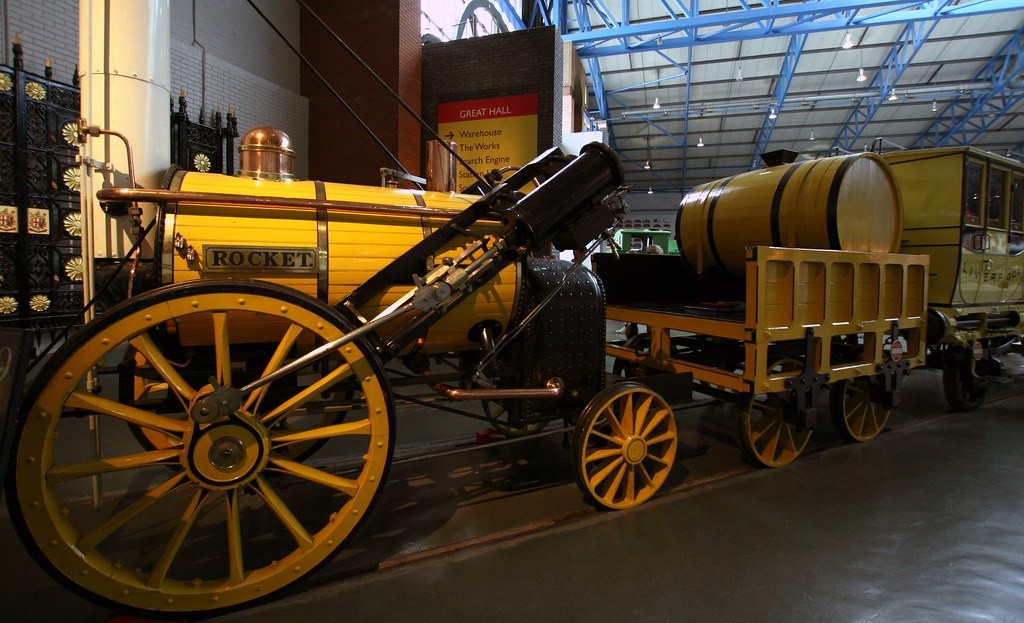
[0,124,1024,617]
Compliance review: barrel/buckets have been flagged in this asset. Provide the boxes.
[674,151,904,284]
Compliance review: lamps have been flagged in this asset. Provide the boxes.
[988,99,995,117]
[888,70,898,100]
[697,133,704,147]
[643,161,651,169]
[929,76,938,112]
[652,96,662,109]
[647,187,653,194]
[856,49,867,82]
[842,29,853,48]
[769,107,777,119]
